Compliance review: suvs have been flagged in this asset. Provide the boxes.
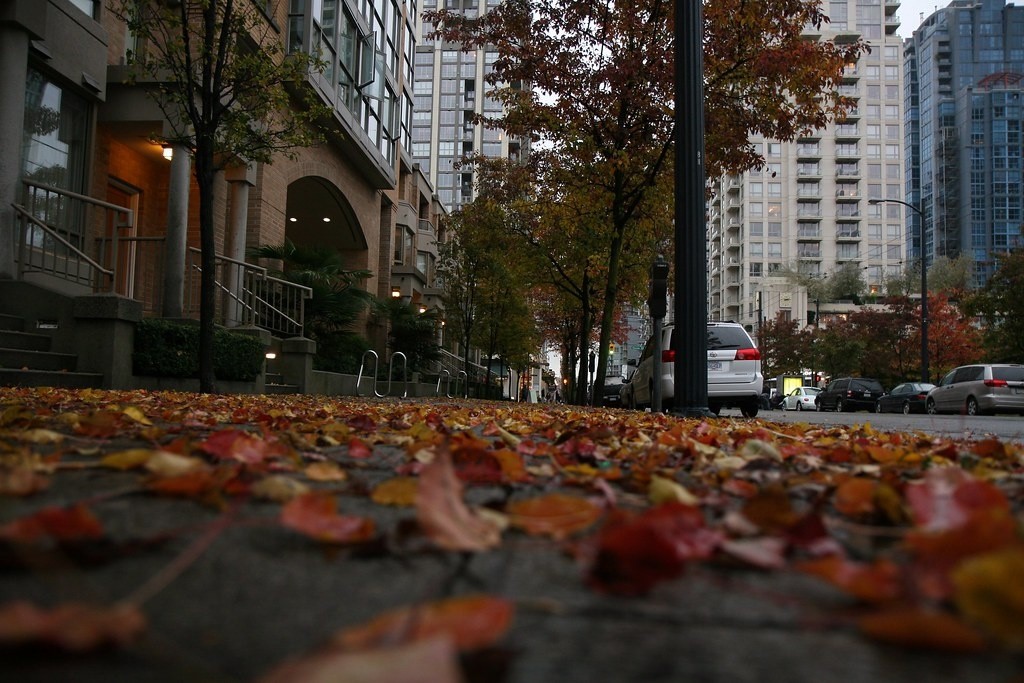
[619,319,764,418]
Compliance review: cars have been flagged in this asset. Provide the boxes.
[815,377,885,413]
[585,375,626,409]
[874,382,937,415]
[924,363,1024,416]
[782,386,824,412]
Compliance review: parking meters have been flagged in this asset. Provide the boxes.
[647,256,669,413]
[589,351,596,408]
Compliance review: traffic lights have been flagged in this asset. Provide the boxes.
[610,344,615,355]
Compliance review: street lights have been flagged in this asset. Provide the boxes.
[868,197,930,383]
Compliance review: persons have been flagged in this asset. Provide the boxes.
[519,385,528,402]
[763,385,770,398]
[546,393,551,404]
